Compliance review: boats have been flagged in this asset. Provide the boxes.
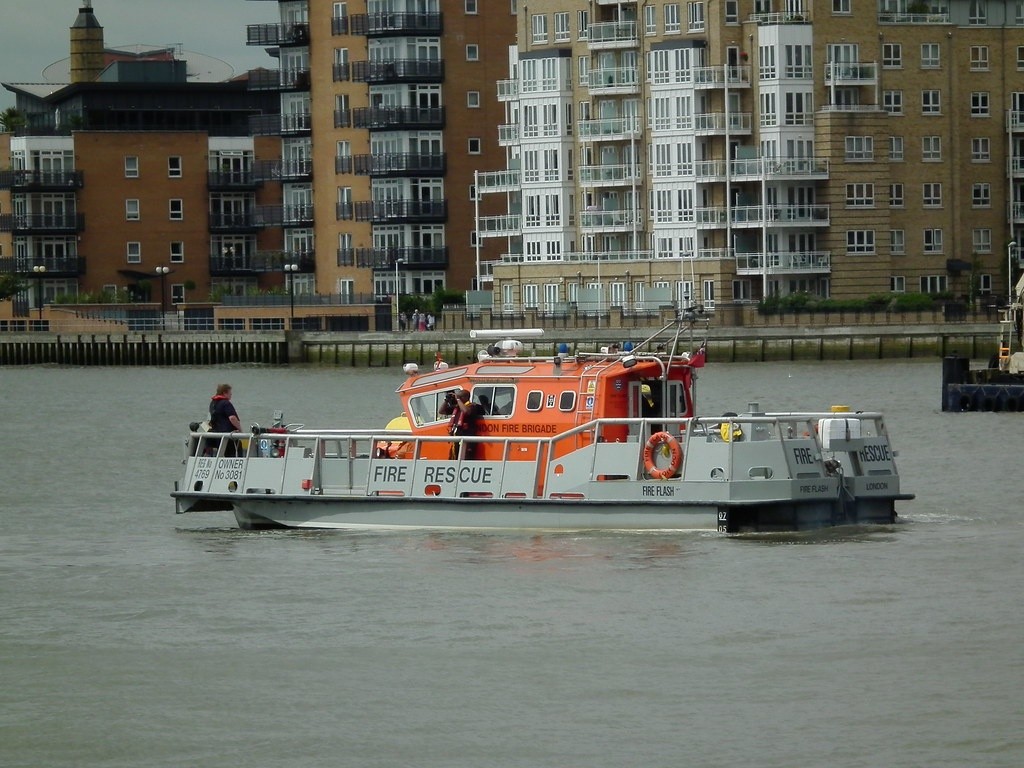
[167,303,924,531]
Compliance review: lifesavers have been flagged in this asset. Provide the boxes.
[647,431,682,479]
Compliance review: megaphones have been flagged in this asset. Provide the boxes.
[487,345,500,357]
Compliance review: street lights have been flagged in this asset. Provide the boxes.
[284,263,299,328]
[34,265,46,319]
[1008,241,1016,323]
[395,258,405,330]
[156,266,171,330]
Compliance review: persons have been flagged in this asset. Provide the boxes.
[209,384,243,458]
[438,390,477,461]
[419,311,426,331]
[426,311,436,331]
[412,309,419,330]
[400,312,407,330]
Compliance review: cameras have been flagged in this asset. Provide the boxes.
[444,394,457,404]
